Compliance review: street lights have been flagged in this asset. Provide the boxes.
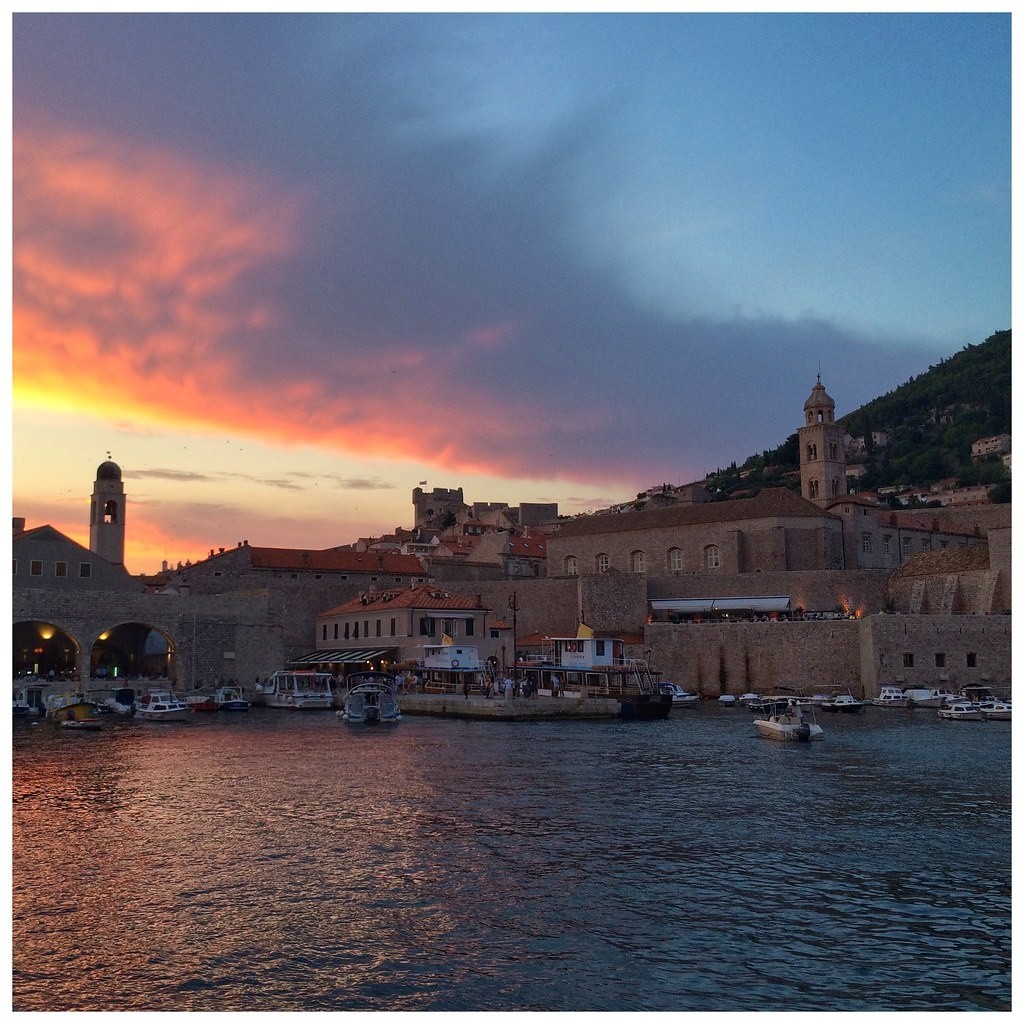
[506,591,520,679]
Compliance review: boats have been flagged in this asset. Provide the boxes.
[653,681,702,708]
[94,697,133,715]
[819,693,865,713]
[420,643,498,696]
[717,694,736,708]
[213,688,249,712]
[337,671,402,725]
[255,670,334,709]
[135,688,191,722]
[872,682,1012,722]
[46,688,105,728]
[181,695,219,712]
[511,637,674,717]
[753,685,824,742]
[738,692,815,713]
[13,681,51,716]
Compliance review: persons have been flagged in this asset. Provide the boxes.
[289,672,423,695]
[254,675,260,687]
[219,677,240,689]
[66,707,75,721]
[463,679,471,699]
[16,667,163,682]
[670,614,842,624]
[479,673,539,700]
[552,675,564,699]
